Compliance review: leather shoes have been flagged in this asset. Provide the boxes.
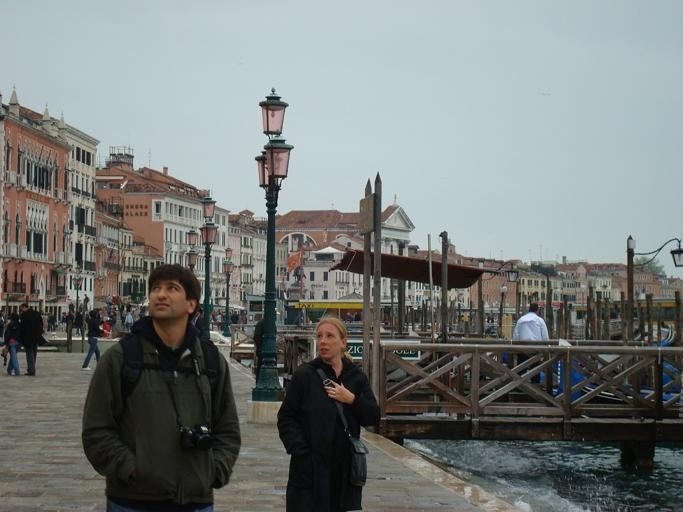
[24,373,35,376]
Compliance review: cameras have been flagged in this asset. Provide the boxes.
[179,424,215,451]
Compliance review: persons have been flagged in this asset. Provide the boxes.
[192,305,245,331]
[81,263,241,512]
[512,303,548,393]
[275,315,379,512]
[0,293,149,377]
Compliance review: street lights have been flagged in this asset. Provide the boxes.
[554,287,562,308]
[494,283,509,333]
[600,282,609,301]
[183,194,232,344]
[72,265,82,312]
[627,235,683,341]
[247,84,296,427]
[576,280,590,331]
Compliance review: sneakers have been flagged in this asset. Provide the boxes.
[81,366,91,370]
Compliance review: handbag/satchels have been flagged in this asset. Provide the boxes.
[338,433,369,488]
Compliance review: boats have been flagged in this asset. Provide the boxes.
[459,314,683,404]
